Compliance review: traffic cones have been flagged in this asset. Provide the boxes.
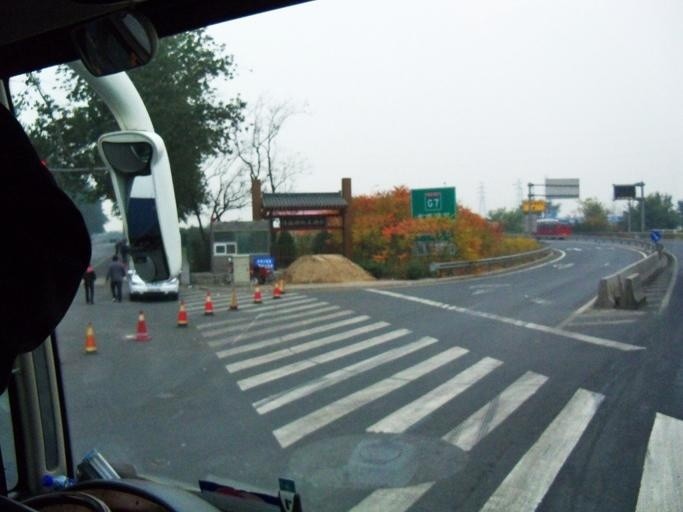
[133,308,152,341]
[273,279,284,299]
[205,292,214,316]
[177,300,188,326]
[253,283,262,303]
[228,286,238,311]
[86,320,97,353]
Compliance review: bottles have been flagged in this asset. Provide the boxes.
[41,474,77,492]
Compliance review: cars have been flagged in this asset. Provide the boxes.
[128,269,180,300]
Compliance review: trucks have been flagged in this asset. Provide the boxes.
[534,219,572,240]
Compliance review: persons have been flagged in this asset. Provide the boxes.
[0,98,92,501]
[106,256,128,303]
[81,265,96,306]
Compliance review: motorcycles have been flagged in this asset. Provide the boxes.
[224,257,265,283]
